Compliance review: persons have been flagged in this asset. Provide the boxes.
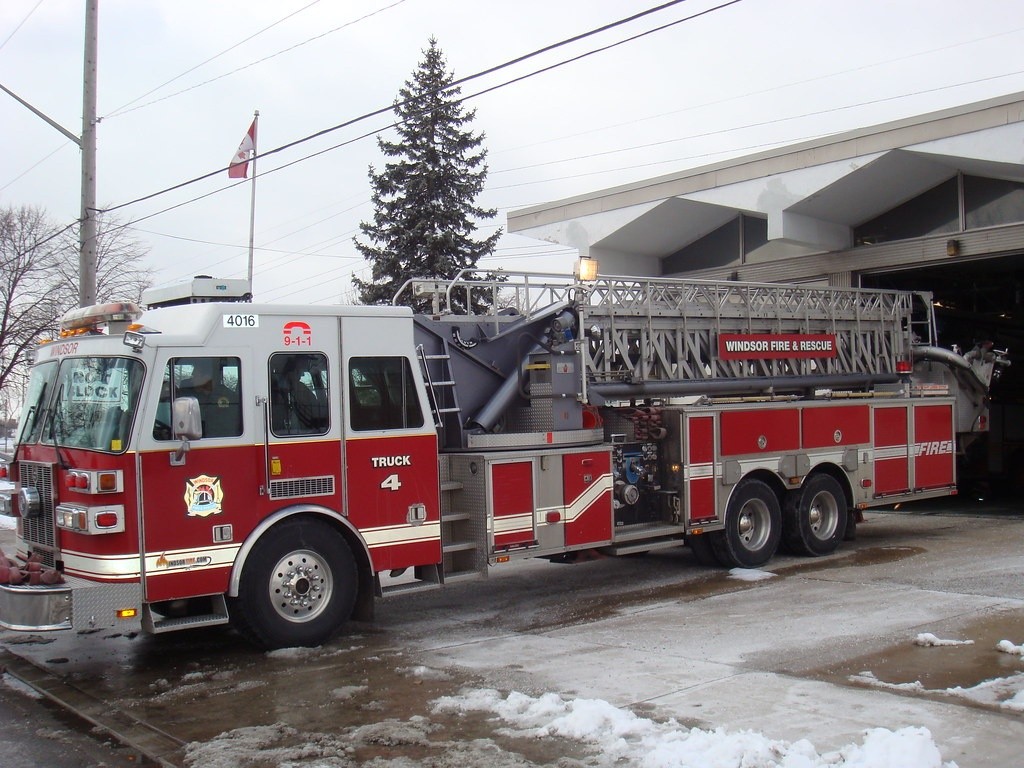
[178,360,241,423]
[284,356,306,381]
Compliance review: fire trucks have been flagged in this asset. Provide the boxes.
[0,256,1012,650]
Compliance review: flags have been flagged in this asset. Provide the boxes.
[228,121,255,178]
